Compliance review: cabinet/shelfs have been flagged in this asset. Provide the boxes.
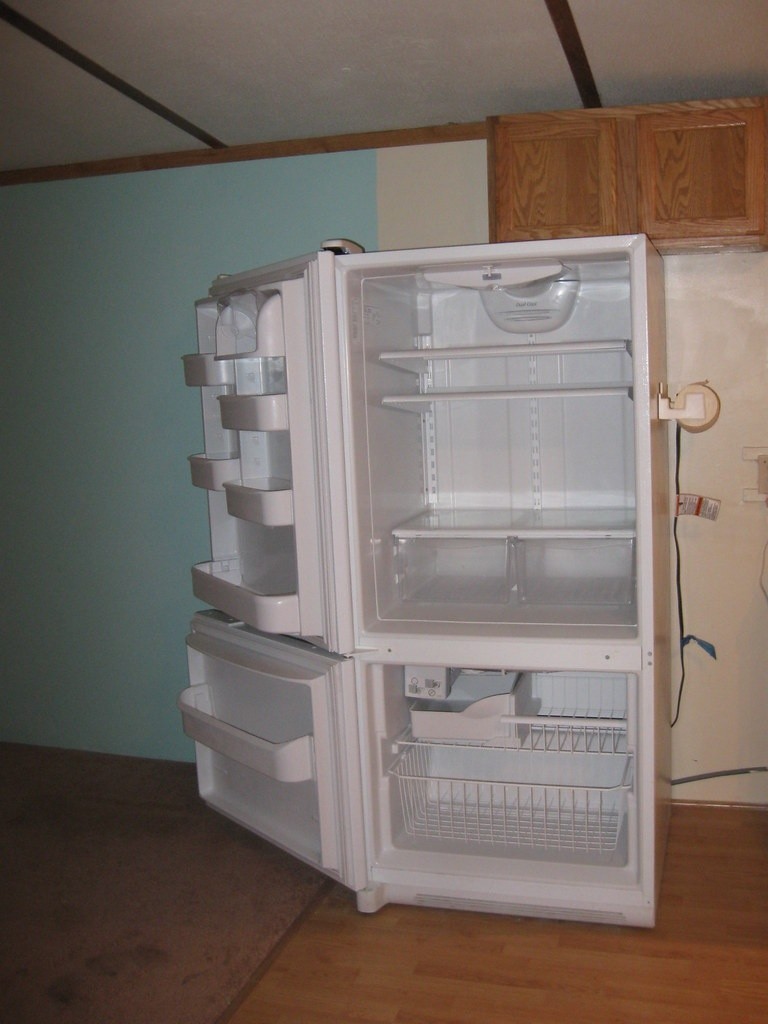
[486,93,768,256]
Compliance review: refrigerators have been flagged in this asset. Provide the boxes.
[180,241,702,927]
